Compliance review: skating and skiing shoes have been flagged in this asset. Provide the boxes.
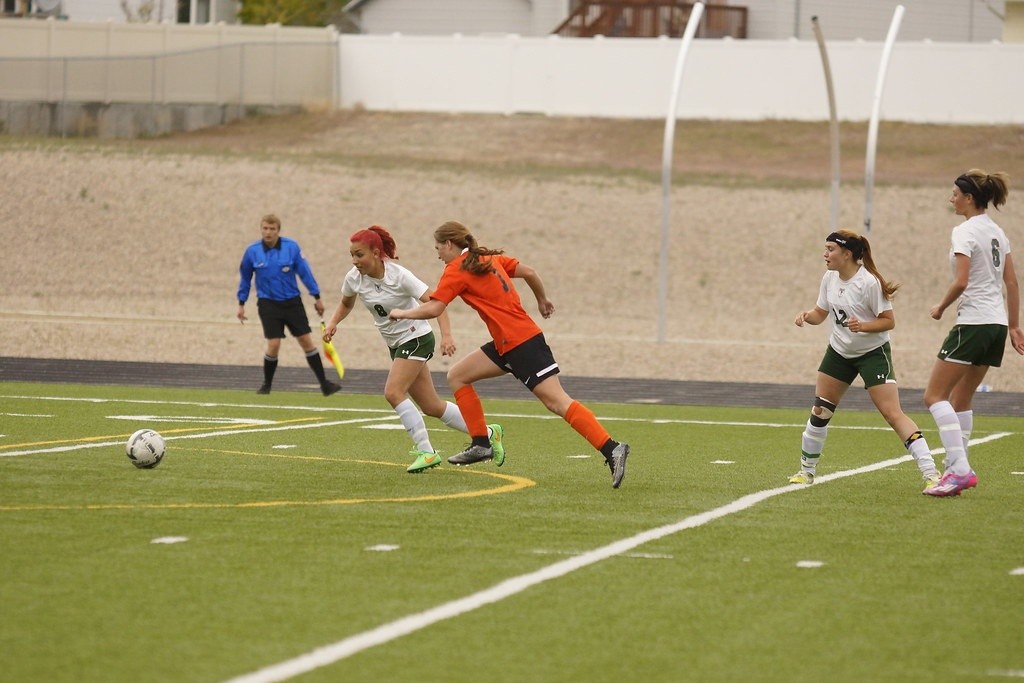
[319,381,341,396]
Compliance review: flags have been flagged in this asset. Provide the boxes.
[320,325,344,379]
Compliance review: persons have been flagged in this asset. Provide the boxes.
[921,168,1024,498]
[321,224,507,474]
[235,213,343,398]
[387,218,631,490]
[788,228,943,496]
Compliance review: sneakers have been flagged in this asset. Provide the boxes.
[486,424,506,467]
[448,443,493,465]
[257,384,271,395]
[790,471,814,484]
[604,442,630,489]
[925,470,976,496]
[922,471,942,495]
[407,446,442,473]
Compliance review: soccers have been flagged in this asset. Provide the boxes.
[125,429,168,471]
